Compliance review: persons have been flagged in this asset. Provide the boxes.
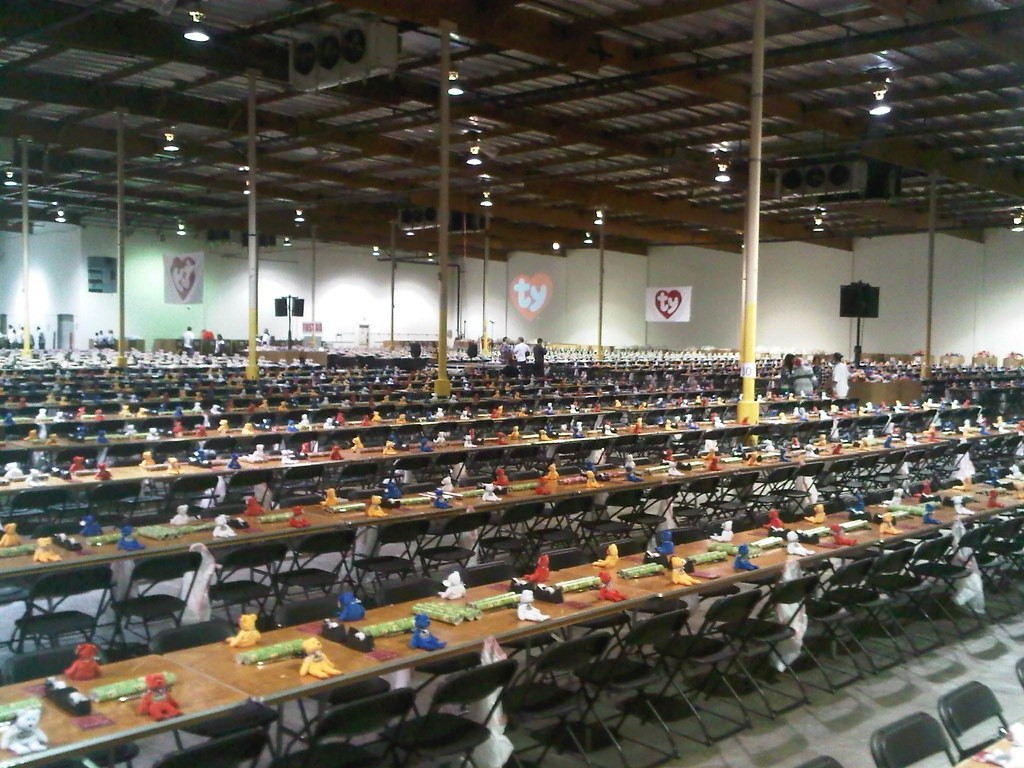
[215,334,225,353]
[183,327,194,358]
[37,327,45,349]
[824,353,850,398]
[499,337,511,354]
[533,338,547,377]
[779,354,794,382]
[813,355,835,398]
[812,353,823,399]
[791,359,818,396]
[260,329,271,346]
[513,337,531,369]
[202,330,214,355]
[270,336,277,346]
[7,324,34,348]
[95,329,114,348]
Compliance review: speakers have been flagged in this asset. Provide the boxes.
[291,298,304,317]
[839,285,880,318]
[275,299,287,317]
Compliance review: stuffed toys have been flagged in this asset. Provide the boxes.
[0,459,1024,756]
[0,366,1024,577]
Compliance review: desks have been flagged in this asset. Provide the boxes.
[1,392,1024,768]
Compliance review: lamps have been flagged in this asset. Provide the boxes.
[866,70,892,114]
[583,231,595,243]
[593,208,605,225]
[1008,209,1024,233]
[163,130,179,151]
[243,179,250,195]
[373,246,380,255]
[177,222,187,237]
[55,209,67,222]
[295,208,305,221]
[3,170,17,185]
[446,71,465,96]
[715,152,732,183]
[480,189,493,207]
[812,209,824,231]
[406,229,415,235]
[283,236,292,246]
[184,6,210,42]
[466,141,481,165]
[428,251,435,261]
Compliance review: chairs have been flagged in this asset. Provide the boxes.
[6,368,1024,768]
